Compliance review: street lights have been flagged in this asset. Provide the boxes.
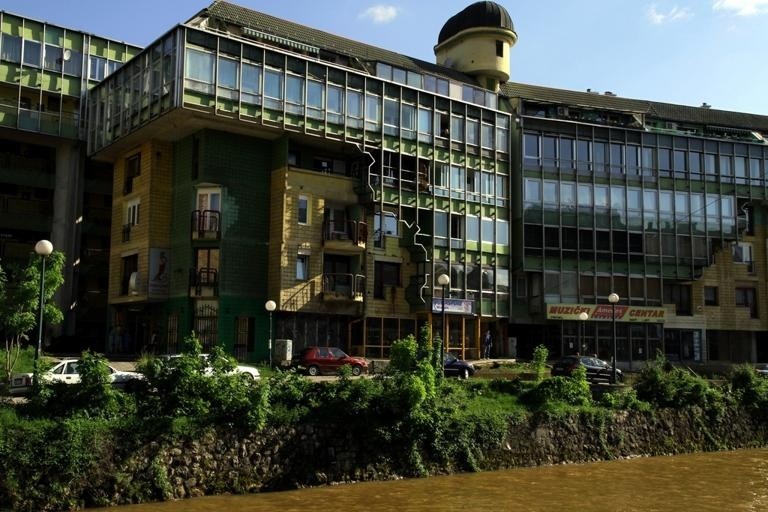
[609,292,620,385]
[579,312,589,354]
[438,273,450,377]
[266,300,277,387]
[30,240,53,400]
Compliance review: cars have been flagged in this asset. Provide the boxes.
[754,363,768,379]
[549,356,624,385]
[10,356,145,396]
[154,354,261,389]
[291,346,370,377]
[386,349,475,378]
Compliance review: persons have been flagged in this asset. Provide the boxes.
[482,329,494,357]
